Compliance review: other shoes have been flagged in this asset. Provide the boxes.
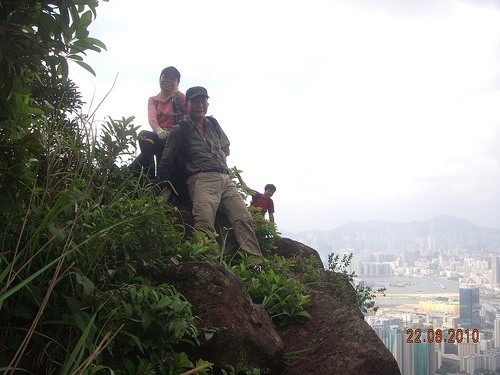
[249,260,265,272]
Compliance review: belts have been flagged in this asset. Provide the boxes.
[186,168,228,175]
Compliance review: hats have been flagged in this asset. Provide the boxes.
[186,86,209,100]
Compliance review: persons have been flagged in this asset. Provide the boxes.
[233,166,277,238]
[165,85,265,274]
[138,66,189,204]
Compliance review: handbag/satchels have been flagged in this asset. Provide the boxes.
[147,159,186,198]
[139,131,155,152]
